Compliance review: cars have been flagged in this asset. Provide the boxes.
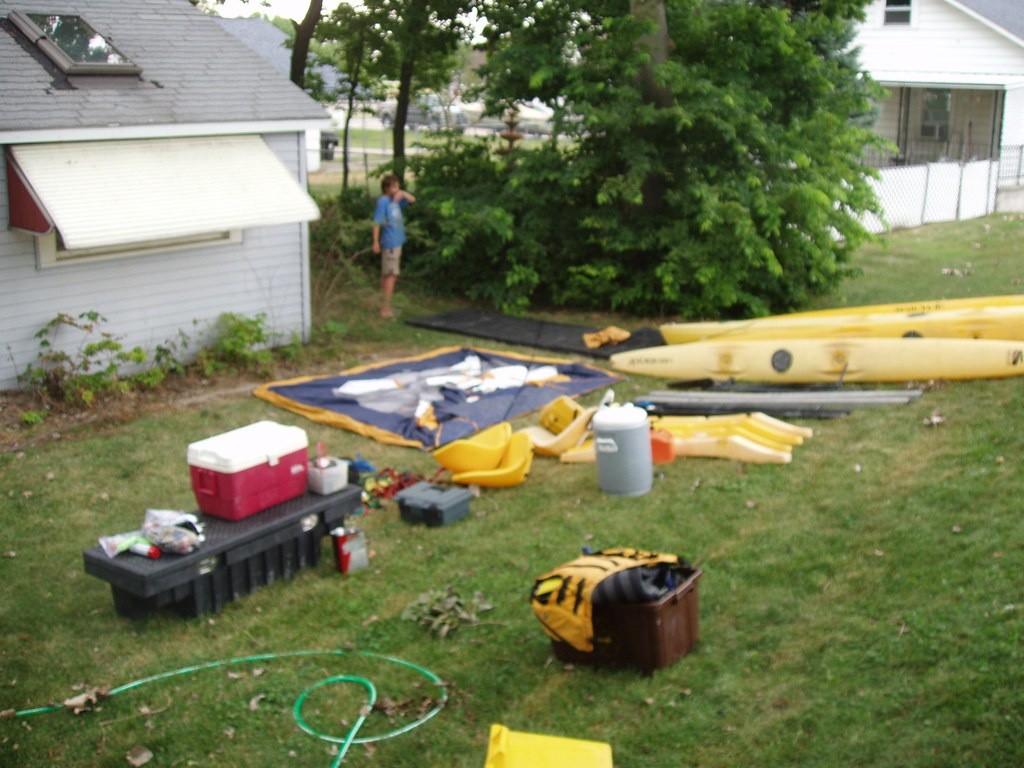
[381,94,463,127]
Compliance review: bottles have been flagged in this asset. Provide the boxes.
[129,544,160,558]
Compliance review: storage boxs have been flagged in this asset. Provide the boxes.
[394,481,475,524]
[187,420,309,522]
[307,456,349,495]
[552,566,703,677]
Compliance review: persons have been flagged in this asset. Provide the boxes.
[328,140,338,159]
[372,170,416,319]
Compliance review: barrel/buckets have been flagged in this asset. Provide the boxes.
[331,525,368,575]
[593,400,652,497]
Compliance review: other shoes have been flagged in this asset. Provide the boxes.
[381,308,394,317]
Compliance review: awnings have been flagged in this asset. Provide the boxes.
[4,134,322,253]
